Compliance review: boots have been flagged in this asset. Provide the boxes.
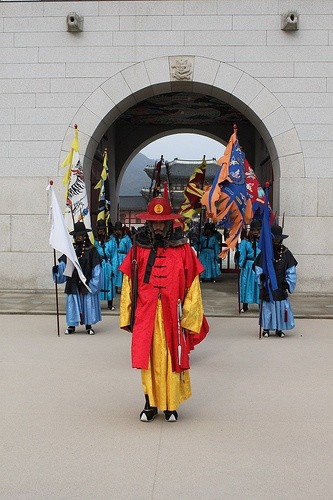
[140,394,157,422]
[108,300,115,311]
[199,279,203,283]
[275,329,285,337]
[263,329,269,337]
[211,278,216,283]
[240,303,249,312]
[64,326,75,335]
[86,325,96,335]
[164,410,178,422]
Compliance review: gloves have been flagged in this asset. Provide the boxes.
[282,282,290,290]
[52,265,59,274]
[260,273,267,283]
[235,251,241,259]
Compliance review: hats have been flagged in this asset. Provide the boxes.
[69,222,93,236]
[96,219,124,230]
[249,219,262,230]
[135,197,183,221]
[202,223,217,230]
[270,224,289,241]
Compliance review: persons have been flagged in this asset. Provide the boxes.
[51,223,103,335]
[252,224,299,337]
[94,221,262,311]
[116,193,210,422]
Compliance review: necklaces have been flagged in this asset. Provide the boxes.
[73,241,86,258]
[147,227,173,257]
[268,245,284,263]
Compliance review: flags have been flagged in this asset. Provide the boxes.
[61,124,260,259]
[49,185,92,294]
[257,187,279,304]
